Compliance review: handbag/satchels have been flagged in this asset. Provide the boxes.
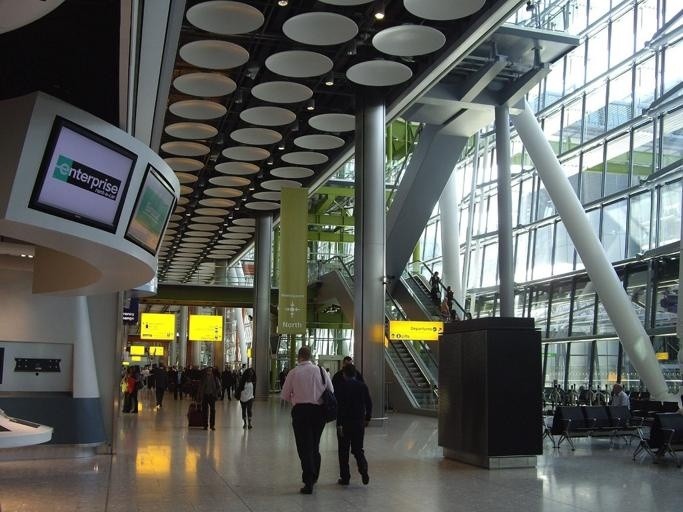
[318,364,338,422]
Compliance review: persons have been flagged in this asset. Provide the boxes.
[611,384,631,422]
[333,357,366,382]
[675,395,683,414]
[428,271,459,322]
[279,368,288,389]
[334,363,372,484]
[281,346,336,494]
[120,361,257,430]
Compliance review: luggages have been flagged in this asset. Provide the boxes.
[187,401,204,426]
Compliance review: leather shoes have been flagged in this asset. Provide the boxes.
[362,472,369,484]
[300,487,312,494]
[338,478,349,485]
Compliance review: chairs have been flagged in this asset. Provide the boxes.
[544,398,682,472]
